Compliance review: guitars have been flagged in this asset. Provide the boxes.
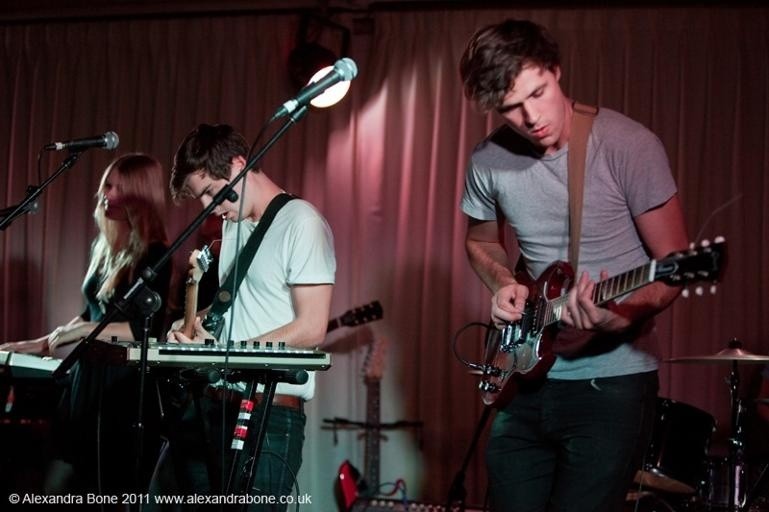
[334,333,406,512]
[134,244,225,440]
[478,237,726,410]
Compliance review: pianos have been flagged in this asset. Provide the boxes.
[1,349,72,381]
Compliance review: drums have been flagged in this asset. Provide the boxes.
[631,398,717,495]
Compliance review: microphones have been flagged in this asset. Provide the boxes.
[274,57,359,120]
[43,130,120,151]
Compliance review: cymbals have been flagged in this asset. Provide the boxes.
[662,347,765,364]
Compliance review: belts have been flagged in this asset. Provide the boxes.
[205,385,304,410]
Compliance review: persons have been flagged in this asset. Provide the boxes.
[146,123,337,512]
[0,153,173,512]
[460,18,691,512]
